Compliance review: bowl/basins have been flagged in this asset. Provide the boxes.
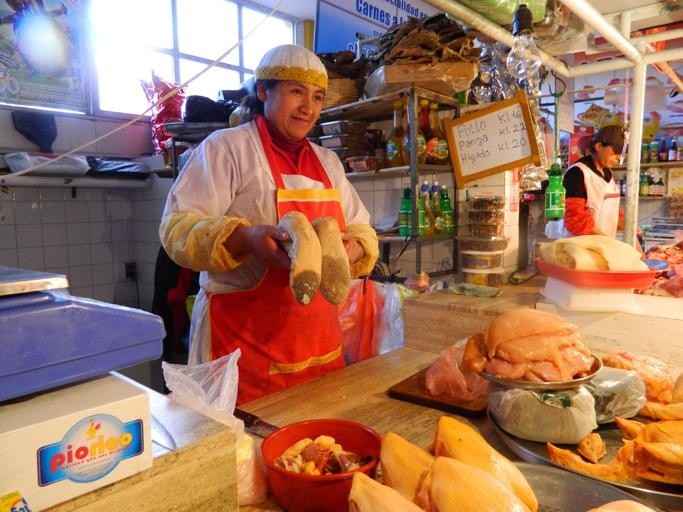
[258,418,384,511]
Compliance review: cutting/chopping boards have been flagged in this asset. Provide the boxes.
[388,366,486,418]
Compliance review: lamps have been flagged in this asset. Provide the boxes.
[506,4,542,80]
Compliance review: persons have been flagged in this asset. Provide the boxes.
[158,45,381,408]
[547,125,627,237]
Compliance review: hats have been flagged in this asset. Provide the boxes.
[592,125,627,144]
[254,44,329,89]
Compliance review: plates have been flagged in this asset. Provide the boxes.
[476,355,604,391]
[535,255,656,290]
[487,415,683,511]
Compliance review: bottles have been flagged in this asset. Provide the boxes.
[419,100,437,165]
[678,147,683,160]
[401,96,426,167]
[642,143,648,163]
[440,184,455,235]
[669,138,677,161]
[659,136,667,161]
[431,180,444,234]
[649,175,656,195]
[421,180,435,236]
[386,100,404,169]
[408,185,425,235]
[430,103,449,165]
[656,178,664,196]
[399,187,413,235]
[622,176,627,195]
[650,141,659,162]
[545,163,566,221]
[640,172,649,196]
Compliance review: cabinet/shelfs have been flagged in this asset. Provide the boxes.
[170,86,462,293]
[561,163,682,200]
